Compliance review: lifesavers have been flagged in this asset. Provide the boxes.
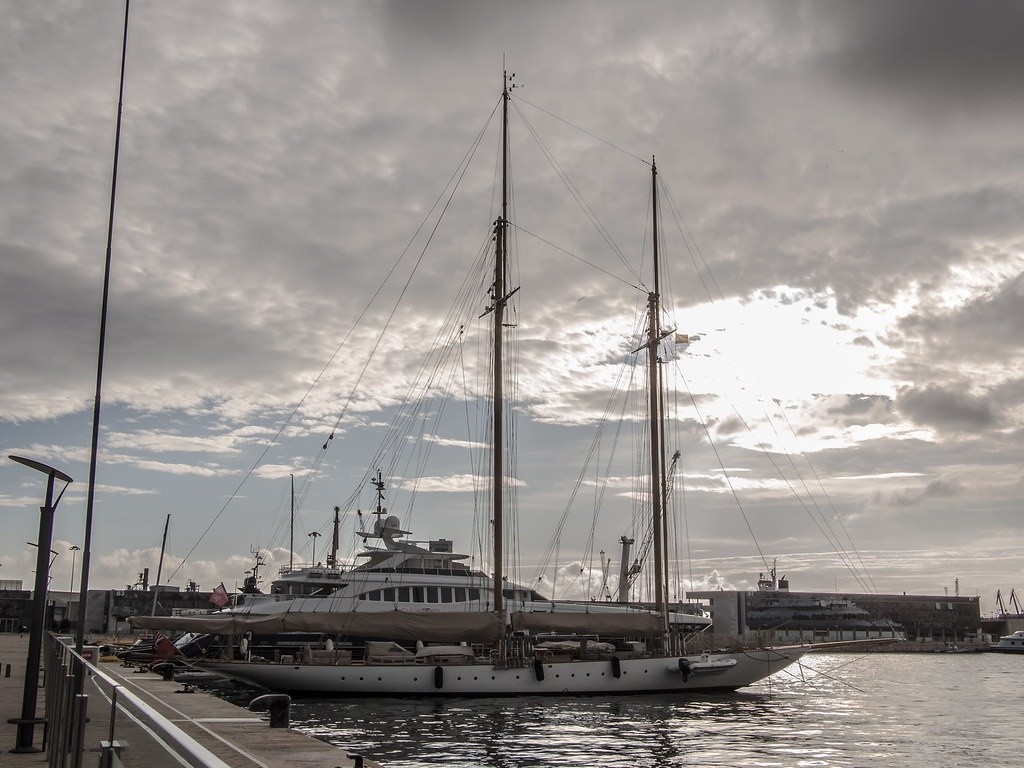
[544,650,554,661]
[489,649,498,661]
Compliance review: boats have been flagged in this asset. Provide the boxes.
[989,628,1024,655]
[115,60,908,696]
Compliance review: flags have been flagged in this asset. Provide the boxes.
[209,585,228,609]
[153,632,172,658]
